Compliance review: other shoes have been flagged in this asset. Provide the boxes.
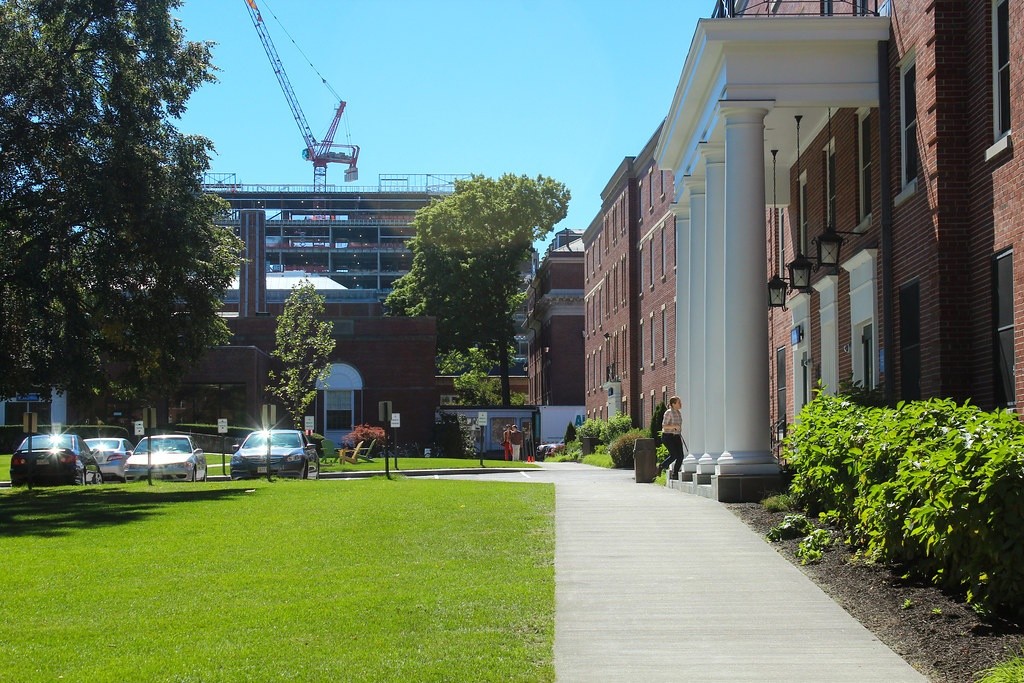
[659,464,663,477]
[671,473,677,479]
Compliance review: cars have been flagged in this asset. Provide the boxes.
[82,437,135,483]
[123,434,208,483]
[10,433,104,486]
[230,429,320,480]
[537,438,565,460]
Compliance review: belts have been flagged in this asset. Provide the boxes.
[663,433,679,436]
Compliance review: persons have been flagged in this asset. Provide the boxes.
[500,423,523,461]
[656,395,683,480]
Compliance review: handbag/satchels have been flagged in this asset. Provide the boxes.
[500,441,505,446]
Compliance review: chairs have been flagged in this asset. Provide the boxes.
[338,441,364,464]
[320,440,341,464]
[354,439,378,464]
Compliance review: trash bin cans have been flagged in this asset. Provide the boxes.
[632,438,657,483]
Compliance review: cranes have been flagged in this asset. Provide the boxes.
[245,0,360,245]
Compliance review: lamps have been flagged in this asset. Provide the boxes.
[785,250,818,294]
[765,274,791,311]
[811,223,868,277]
